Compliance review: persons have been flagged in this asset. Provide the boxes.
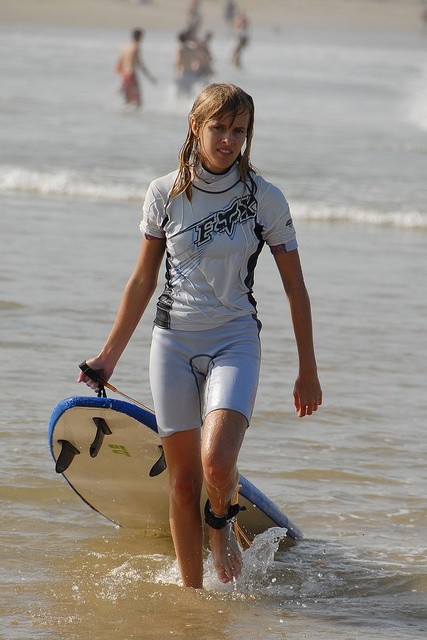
[78,84,324,594]
[101,2,251,111]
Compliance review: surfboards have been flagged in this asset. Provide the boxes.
[48,397,305,539]
